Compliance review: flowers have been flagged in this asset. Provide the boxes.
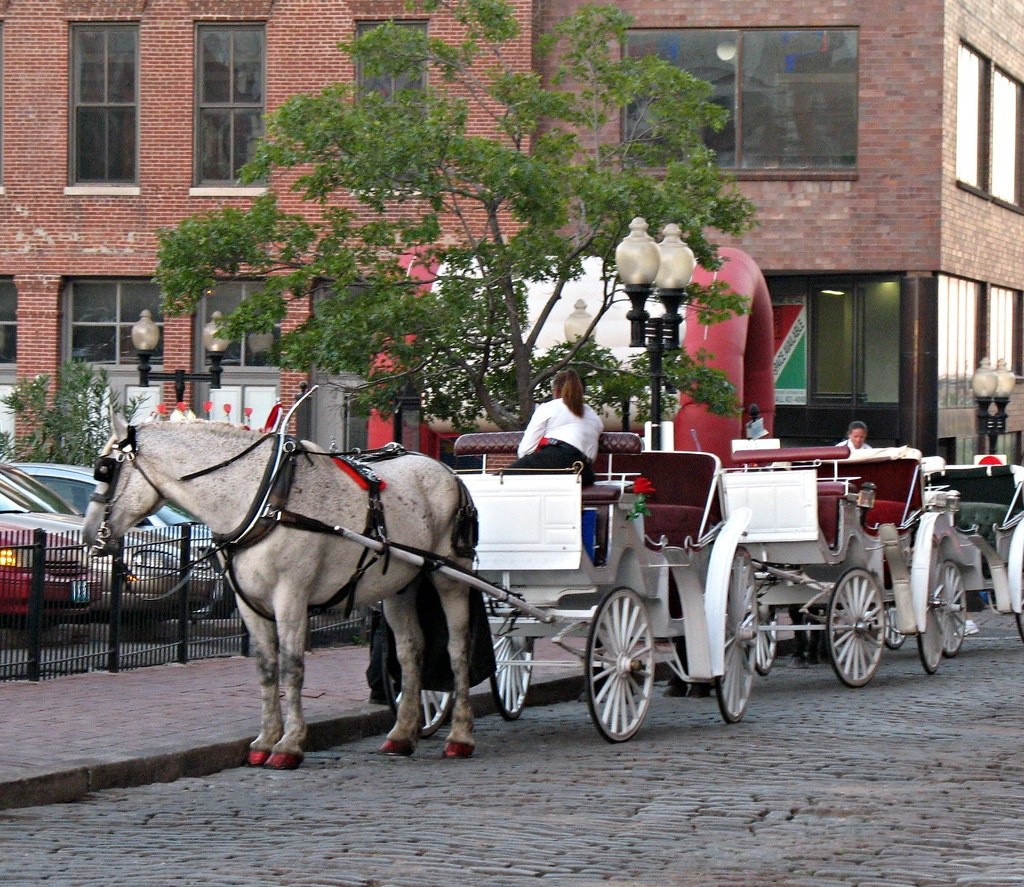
[627,477,657,521]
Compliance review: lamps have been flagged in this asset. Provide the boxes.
[946,490,962,525]
[857,482,878,527]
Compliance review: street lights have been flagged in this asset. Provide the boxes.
[616,214,701,452]
[133,305,231,660]
[972,356,1016,455]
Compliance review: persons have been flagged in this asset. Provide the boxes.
[835,421,873,449]
[493,368,604,487]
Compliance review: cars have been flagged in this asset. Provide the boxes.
[0,461,221,618]
[8,462,243,624]
[0,524,104,628]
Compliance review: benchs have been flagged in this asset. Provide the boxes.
[454,432,1024,557]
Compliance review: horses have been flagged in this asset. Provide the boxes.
[82,404,479,770]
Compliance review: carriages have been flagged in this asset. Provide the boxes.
[74,410,1024,772]
